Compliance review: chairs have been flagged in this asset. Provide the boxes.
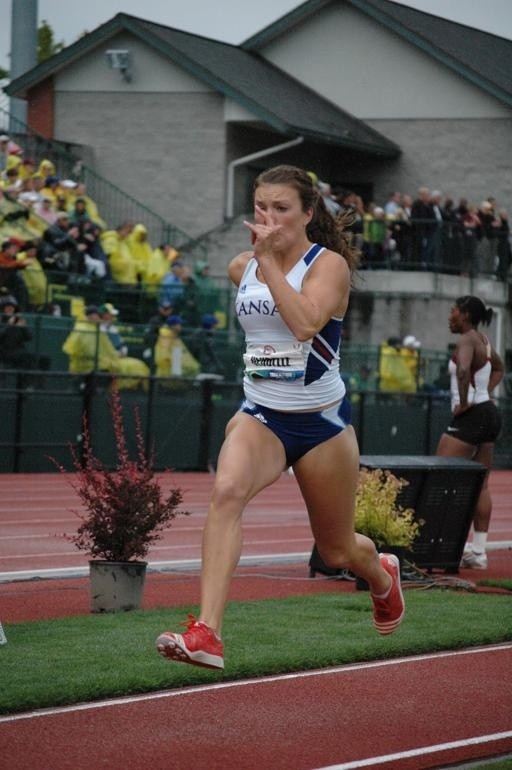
[0,139,244,383]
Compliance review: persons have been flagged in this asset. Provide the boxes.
[2,133,215,321]
[157,162,408,671]
[438,295,504,572]
[399,336,422,391]
[153,316,201,375]
[0,295,32,374]
[188,315,234,381]
[379,336,413,392]
[144,297,175,341]
[60,304,127,393]
[97,301,152,370]
[348,361,373,401]
[308,167,512,282]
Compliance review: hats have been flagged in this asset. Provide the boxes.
[84,303,120,316]
[403,336,421,349]
[158,299,219,325]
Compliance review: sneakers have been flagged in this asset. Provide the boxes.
[370,553,406,636]
[458,550,487,569]
[155,613,225,669]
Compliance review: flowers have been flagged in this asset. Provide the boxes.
[349,464,424,554]
[49,371,199,564]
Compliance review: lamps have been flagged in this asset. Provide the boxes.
[107,48,136,84]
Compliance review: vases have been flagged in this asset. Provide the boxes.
[87,554,150,614]
[356,544,408,594]
